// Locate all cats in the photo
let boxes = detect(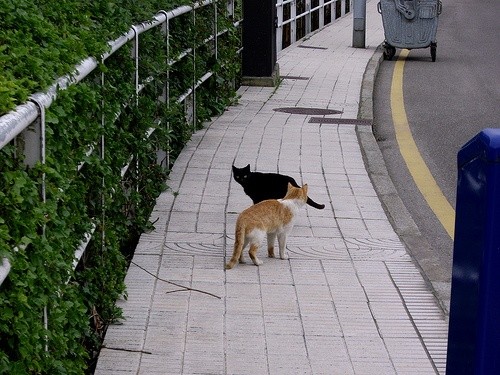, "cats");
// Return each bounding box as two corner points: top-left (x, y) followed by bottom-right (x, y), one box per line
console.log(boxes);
(225, 181), (308, 270)
(232, 164), (325, 210)
